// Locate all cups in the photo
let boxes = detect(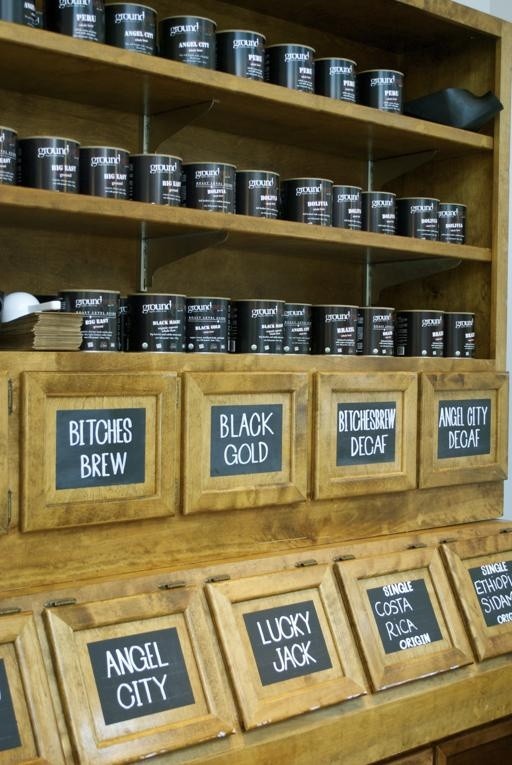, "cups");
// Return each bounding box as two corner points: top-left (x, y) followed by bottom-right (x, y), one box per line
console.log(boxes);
(160, 15), (219, 72)
(0, 126), (465, 249)
(214, 28), (267, 82)
(357, 69), (409, 116)
(1, 286), (479, 361)
(314, 56), (357, 104)
(0, 0), (159, 57)
(265, 40), (316, 93)
